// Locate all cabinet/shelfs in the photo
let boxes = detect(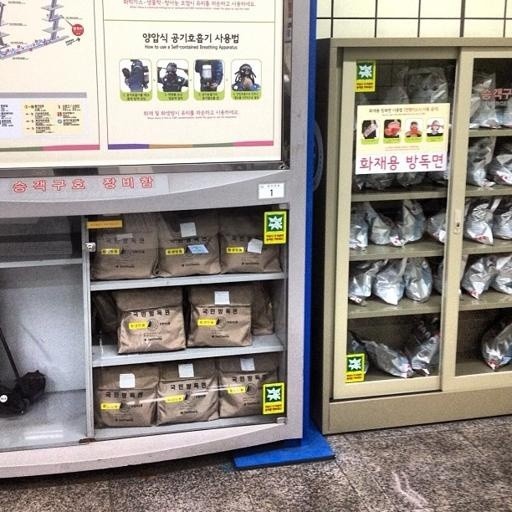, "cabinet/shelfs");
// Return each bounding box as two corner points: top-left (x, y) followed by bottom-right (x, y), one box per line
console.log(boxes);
(0, 189), (305, 481)
(316, 36), (512, 432)
